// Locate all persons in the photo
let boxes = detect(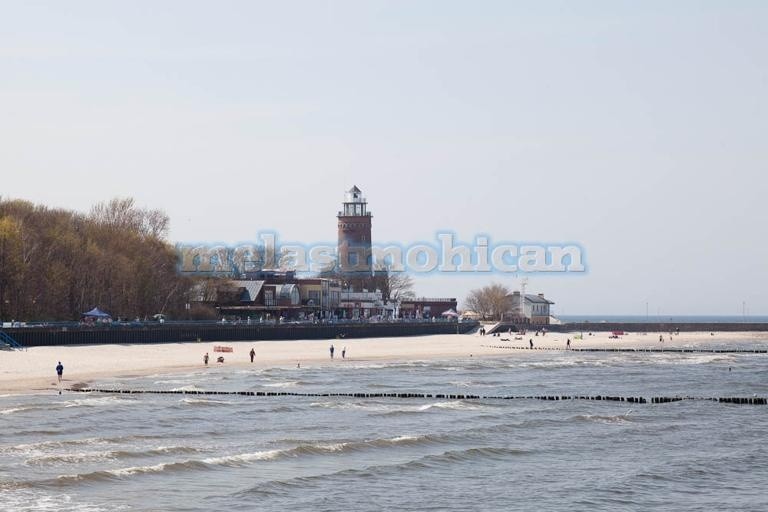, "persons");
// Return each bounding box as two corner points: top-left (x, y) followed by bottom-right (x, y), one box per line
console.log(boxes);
(55, 361), (63, 383)
(219, 309), (475, 326)
(81, 314), (165, 330)
(328, 343), (335, 360)
(248, 348), (256, 363)
(202, 352), (208, 366)
